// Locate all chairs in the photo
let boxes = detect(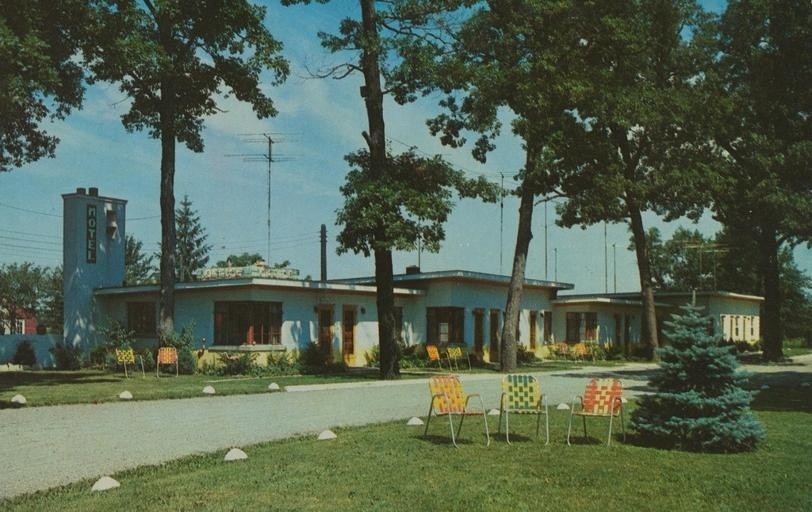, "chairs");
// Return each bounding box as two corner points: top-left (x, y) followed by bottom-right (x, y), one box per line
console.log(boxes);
(567, 377), (625, 449)
(113, 344), (147, 378)
(498, 374), (551, 448)
(155, 347), (179, 379)
(418, 342), (617, 371)
(424, 374), (491, 451)
